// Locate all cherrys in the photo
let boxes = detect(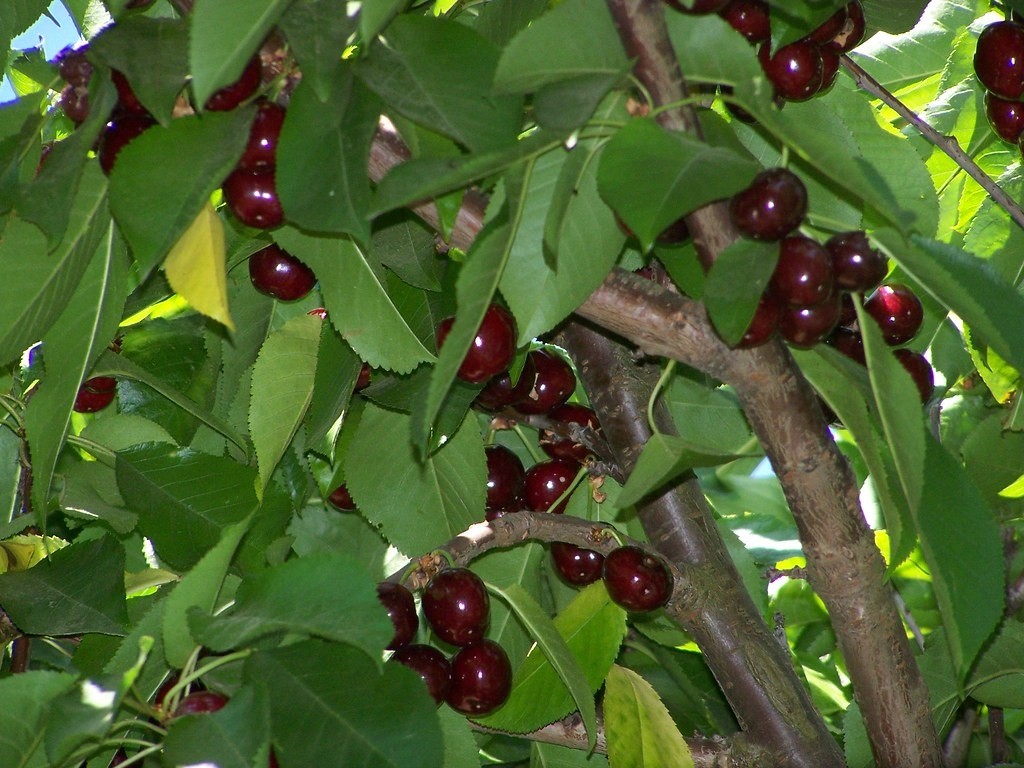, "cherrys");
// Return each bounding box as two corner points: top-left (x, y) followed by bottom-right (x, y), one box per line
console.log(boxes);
(64, 0), (1024, 727)
